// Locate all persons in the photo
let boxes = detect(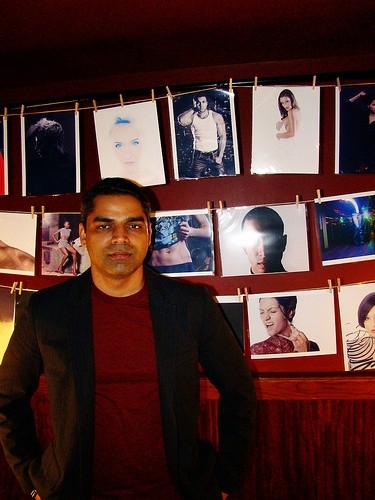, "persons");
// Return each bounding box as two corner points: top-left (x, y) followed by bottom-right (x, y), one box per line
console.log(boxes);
(344, 294), (374, 367)
(111, 117), (145, 181)
(177, 95), (227, 176)
(268, 88), (302, 159)
(52, 221), (79, 275)
(240, 206), (286, 274)
(27, 118), (65, 187)
(0, 177), (256, 500)
(349, 91), (375, 172)
(252, 297), (320, 354)
(147, 215), (210, 274)
